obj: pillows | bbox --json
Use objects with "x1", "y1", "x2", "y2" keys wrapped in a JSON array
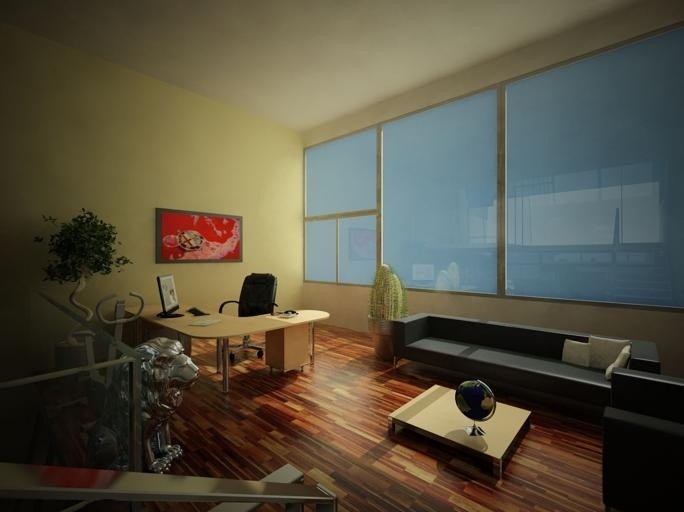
[
  {"x1": 588, "y1": 335, "x2": 631, "y2": 370},
  {"x1": 605, "y1": 341, "x2": 632, "y2": 380},
  {"x1": 561, "y1": 337, "x2": 592, "y2": 368}
]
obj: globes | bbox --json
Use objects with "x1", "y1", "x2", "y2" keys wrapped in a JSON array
[{"x1": 455, "y1": 379, "x2": 496, "y2": 436}]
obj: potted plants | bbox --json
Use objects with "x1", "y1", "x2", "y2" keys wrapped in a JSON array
[
  {"x1": 367, "y1": 264, "x2": 408, "y2": 362},
  {"x1": 33, "y1": 206, "x2": 135, "y2": 385}
]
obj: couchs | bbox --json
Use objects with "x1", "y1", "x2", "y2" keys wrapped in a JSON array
[{"x1": 392, "y1": 312, "x2": 660, "y2": 408}]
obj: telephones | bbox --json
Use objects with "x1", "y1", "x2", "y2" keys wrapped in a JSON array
[{"x1": 277, "y1": 310, "x2": 299, "y2": 318}]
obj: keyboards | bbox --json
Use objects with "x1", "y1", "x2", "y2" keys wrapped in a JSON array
[{"x1": 189, "y1": 308, "x2": 205, "y2": 316}]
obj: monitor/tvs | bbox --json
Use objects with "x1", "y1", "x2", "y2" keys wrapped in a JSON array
[{"x1": 157, "y1": 273, "x2": 184, "y2": 318}]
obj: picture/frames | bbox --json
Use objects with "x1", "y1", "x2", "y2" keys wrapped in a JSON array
[{"x1": 156, "y1": 208, "x2": 243, "y2": 263}]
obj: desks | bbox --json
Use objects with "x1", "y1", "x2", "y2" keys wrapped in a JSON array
[{"x1": 125, "y1": 302, "x2": 330, "y2": 394}]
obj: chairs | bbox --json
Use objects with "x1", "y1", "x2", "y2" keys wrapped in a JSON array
[
  {"x1": 219, "y1": 274, "x2": 277, "y2": 363},
  {"x1": 601, "y1": 366, "x2": 684, "y2": 512}
]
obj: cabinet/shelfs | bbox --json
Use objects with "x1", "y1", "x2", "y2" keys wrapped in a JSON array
[{"x1": 265, "y1": 322, "x2": 309, "y2": 378}]
